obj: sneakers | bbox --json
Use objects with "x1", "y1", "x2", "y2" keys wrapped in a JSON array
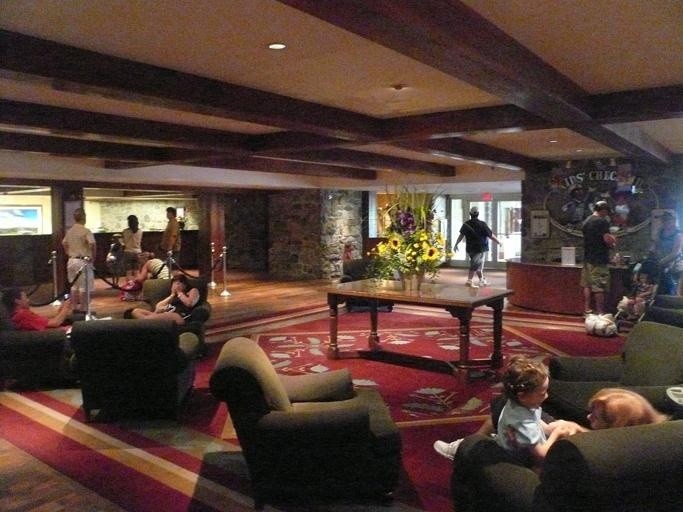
[
  {"x1": 432, "y1": 438, "x2": 465, "y2": 461},
  {"x1": 72, "y1": 309, "x2": 97, "y2": 316},
  {"x1": 583, "y1": 310, "x2": 594, "y2": 319},
  {"x1": 464, "y1": 280, "x2": 490, "y2": 289}
]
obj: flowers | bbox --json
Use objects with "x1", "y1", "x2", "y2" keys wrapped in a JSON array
[{"x1": 360, "y1": 180, "x2": 456, "y2": 283}]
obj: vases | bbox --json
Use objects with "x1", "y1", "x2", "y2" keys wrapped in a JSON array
[{"x1": 396, "y1": 268, "x2": 425, "y2": 291}]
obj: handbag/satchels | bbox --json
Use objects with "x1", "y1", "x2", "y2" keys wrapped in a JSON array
[{"x1": 584, "y1": 313, "x2": 616, "y2": 337}]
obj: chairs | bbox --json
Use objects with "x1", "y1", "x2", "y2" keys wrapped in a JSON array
[
  {"x1": 208, "y1": 336, "x2": 403, "y2": 511},
  {"x1": 0, "y1": 290, "x2": 93, "y2": 389},
  {"x1": 647, "y1": 293, "x2": 682, "y2": 328},
  {"x1": 123, "y1": 275, "x2": 213, "y2": 359},
  {"x1": 541, "y1": 318, "x2": 682, "y2": 429},
  {"x1": 338, "y1": 258, "x2": 394, "y2": 313},
  {"x1": 449, "y1": 416, "x2": 682, "y2": 511},
  {"x1": 69, "y1": 317, "x2": 200, "y2": 429}
]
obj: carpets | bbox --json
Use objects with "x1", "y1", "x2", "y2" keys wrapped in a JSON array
[{"x1": 0, "y1": 299, "x2": 628, "y2": 511}]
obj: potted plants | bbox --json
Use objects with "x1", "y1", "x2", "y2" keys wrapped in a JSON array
[{"x1": 175, "y1": 214, "x2": 187, "y2": 230}]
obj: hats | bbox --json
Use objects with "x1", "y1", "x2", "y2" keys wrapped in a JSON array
[{"x1": 469, "y1": 207, "x2": 479, "y2": 215}]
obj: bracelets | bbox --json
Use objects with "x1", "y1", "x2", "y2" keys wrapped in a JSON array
[{"x1": 177, "y1": 293, "x2": 185, "y2": 297}]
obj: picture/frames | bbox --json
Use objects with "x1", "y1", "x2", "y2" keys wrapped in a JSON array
[{"x1": 0, "y1": 203, "x2": 44, "y2": 236}]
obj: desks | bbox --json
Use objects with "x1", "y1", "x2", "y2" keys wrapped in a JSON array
[{"x1": 314, "y1": 276, "x2": 515, "y2": 384}]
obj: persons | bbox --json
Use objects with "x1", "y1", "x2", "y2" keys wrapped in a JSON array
[
  {"x1": 6, "y1": 287, "x2": 84, "y2": 331},
  {"x1": 123, "y1": 214, "x2": 143, "y2": 283},
  {"x1": 580, "y1": 198, "x2": 616, "y2": 319},
  {"x1": 134, "y1": 249, "x2": 174, "y2": 283},
  {"x1": 454, "y1": 206, "x2": 502, "y2": 286},
  {"x1": 161, "y1": 207, "x2": 182, "y2": 277},
  {"x1": 650, "y1": 211, "x2": 683, "y2": 296},
  {"x1": 434, "y1": 388, "x2": 666, "y2": 470},
  {"x1": 61, "y1": 208, "x2": 97, "y2": 315},
  {"x1": 132, "y1": 274, "x2": 201, "y2": 325},
  {"x1": 498, "y1": 354, "x2": 570, "y2": 466},
  {"x1": 106, "y1": 234, "x2": 124, "y2": 287},
  {"x1": 625, "y1": 268, "x2": 653, "y2": 320}
]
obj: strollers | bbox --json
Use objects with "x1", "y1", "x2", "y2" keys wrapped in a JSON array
[{"x1": 610, "y1": 254, "x2": 676, "y2": 333}]
